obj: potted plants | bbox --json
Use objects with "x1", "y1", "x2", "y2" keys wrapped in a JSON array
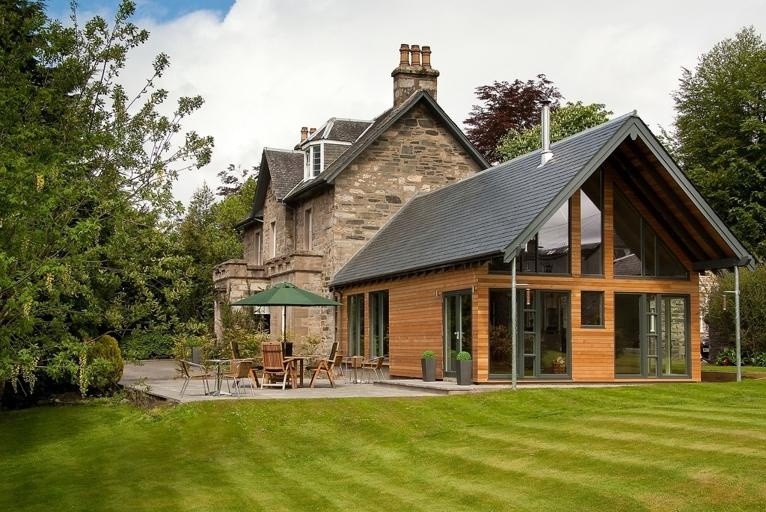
[
  {"x1": 421, "y1": 351, "x2": 437, "y2": 382},
  {"x1": 456, "y1": 352, "x2": 472, "y2": 385}
]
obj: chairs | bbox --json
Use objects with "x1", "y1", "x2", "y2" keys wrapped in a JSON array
[{"x1": 177, "y1": 342, "x2": 385, "y2": 399}]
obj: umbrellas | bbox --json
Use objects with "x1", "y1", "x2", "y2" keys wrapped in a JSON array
[{"x1": 227, "y1": 281, "x2": 345, "y2": 357}]
